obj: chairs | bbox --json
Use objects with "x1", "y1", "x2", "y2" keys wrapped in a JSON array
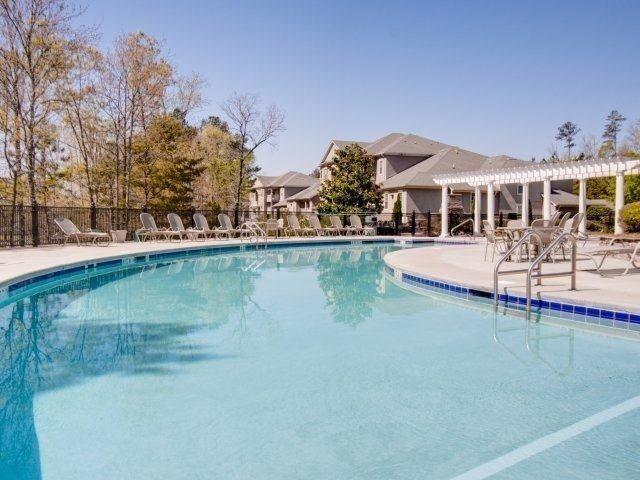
[
  {"x1": 132, "y1": 212, "x2": 378, "y2": 245},
  {"x1": 481, "y1": 209, "x2": 588, "y2": 278},
  {"x1": 49, "y1": 215, "x2": 112, "y2": 245}
]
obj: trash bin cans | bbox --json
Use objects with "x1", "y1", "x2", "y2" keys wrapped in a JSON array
[{"x1": 110, "y1": 230, "x2": 127, "y2": 243}]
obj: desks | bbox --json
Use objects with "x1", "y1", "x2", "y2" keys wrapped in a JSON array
[{"x1": 597, "y1": 232, "x2": 639, "y2": 276}]
